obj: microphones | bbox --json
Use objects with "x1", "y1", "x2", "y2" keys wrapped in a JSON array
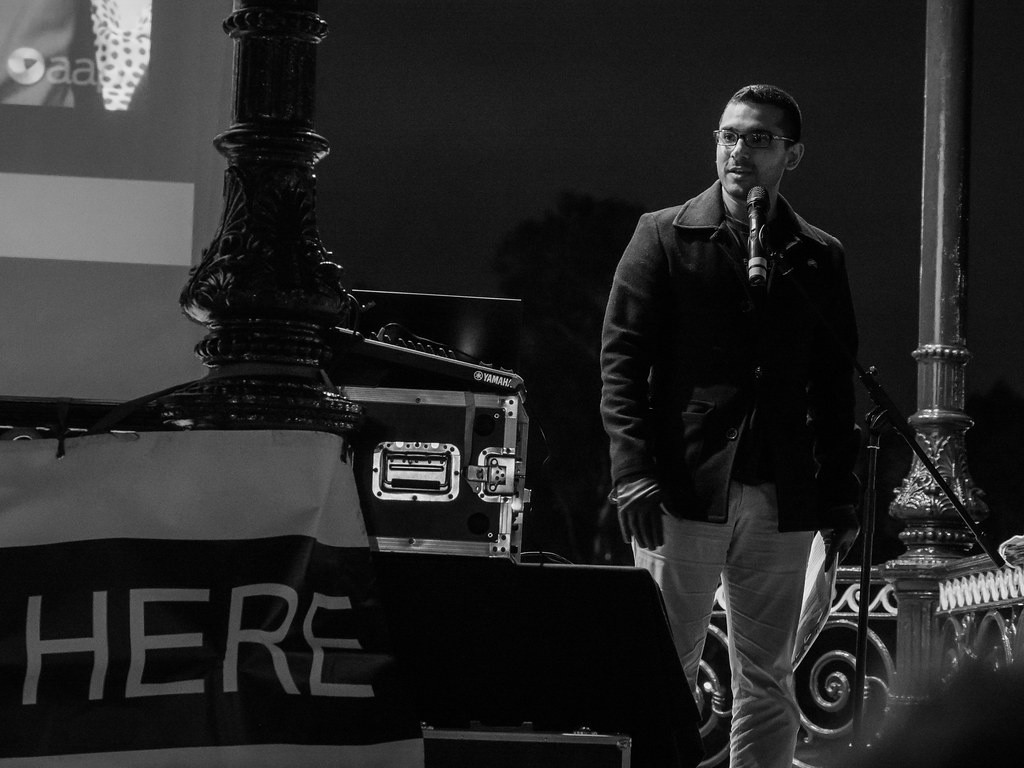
[{"x1": 745, "y1": 186, "x2": 771, "y2": 286}]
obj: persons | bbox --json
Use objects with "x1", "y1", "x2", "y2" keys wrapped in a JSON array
[{"x1": 599, "y1": 84, "x2": 862, "y2": 768}]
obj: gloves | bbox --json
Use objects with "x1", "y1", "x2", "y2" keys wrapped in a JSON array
[
  {"x1": 615, "y1": 473, "x2": 665, "y2": 551},
  {"x1": 813, "y1": 458, "x2": 863, "y2": 529}
]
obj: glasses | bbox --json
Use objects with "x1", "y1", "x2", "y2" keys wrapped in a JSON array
[{"x1": 712, "y1": 130, "x2": 796, "y2": 148}]
